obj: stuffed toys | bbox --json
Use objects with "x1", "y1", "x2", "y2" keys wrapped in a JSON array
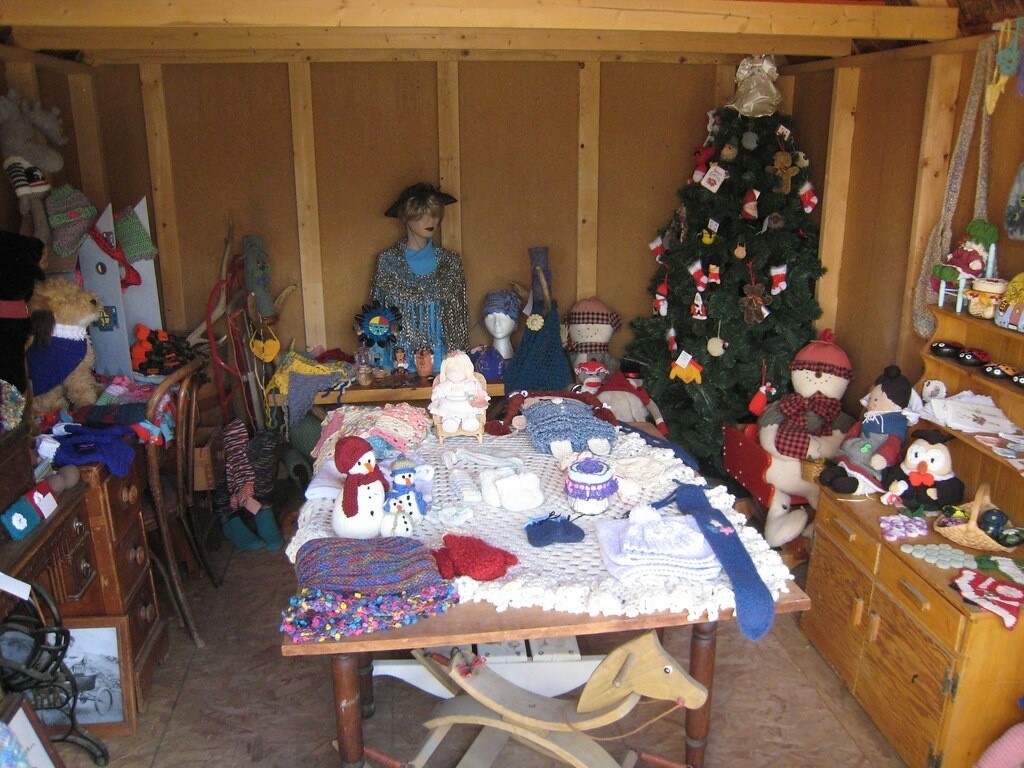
[
  {"x1": 330, "y1": 435, "x2": 426, "y2": 540},
  {"x1": 0, "y1": 96, "x2": 106, "y2": 496},
  {"x1": 560, "y1": 297, "x2": 622, "y2": 396},
  {"x1": 757, "y1": 331, "x2": 965, "y2": 548}
]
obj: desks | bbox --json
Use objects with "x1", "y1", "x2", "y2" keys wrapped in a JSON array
[
  {"x1": 280, "y1": 414, "x2": 812, "y2": 768},
  {"x1": 266, "y1": 373, "x2": 509, "y2": 426}
]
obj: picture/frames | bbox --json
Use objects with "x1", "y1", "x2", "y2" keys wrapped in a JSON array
[
  {"x1": 33, "y1": 611, "x2": 136, "y2": 737},
  {"x1": 2, "y1": 691, "x2": 68, "y2": 768}
]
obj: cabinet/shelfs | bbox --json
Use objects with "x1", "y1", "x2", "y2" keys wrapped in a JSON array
[
  {"x1": 1, "y1": 480, "x2": 108, "y2": 767},
  {"x1": 797, "y1": 302, "x2": 1024, "y2": 768},
  {"x1": 38, "y1": 429, "x2": 171, "y2": 716}
]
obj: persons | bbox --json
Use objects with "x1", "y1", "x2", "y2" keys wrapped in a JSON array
[
  {"x1": 428, "y1": 352, "x2": 491, "y2": 432},
  {"x1": 930, "y1": 219, "x2": 997, "y2": 281},
  {"x1": 369, "y1": 183, "x2": 471, "y2": 372},
  {"x1": 481, "y1": 290, "x2": 522, "y2": 359},
  {"x1": 392, "y1": 345, "x2": 410, "y2": 380}
]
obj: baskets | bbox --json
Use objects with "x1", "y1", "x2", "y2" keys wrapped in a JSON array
[{"x1": 932, "y1": 482, "x2": 1018, "y2": 554}]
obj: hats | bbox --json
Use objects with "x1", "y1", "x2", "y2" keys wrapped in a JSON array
[
  {"x1": 384, "y1": 183, "x2": 457, "y2": 218},
  {"x1": 482, "y1": 289, "x2": 521, "y2": 332}
]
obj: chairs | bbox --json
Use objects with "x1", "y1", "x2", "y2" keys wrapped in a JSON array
[{"x1": 141, "y1": 359, "x2": 222, "y2": 651}]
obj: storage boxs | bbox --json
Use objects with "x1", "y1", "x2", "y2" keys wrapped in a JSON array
[
  {"x1": 0, "y1": 422, "x2": 38, "y2": 518},
  {"x1": 187, "y1": 424, "x2": 222, "y2": 490}
]
